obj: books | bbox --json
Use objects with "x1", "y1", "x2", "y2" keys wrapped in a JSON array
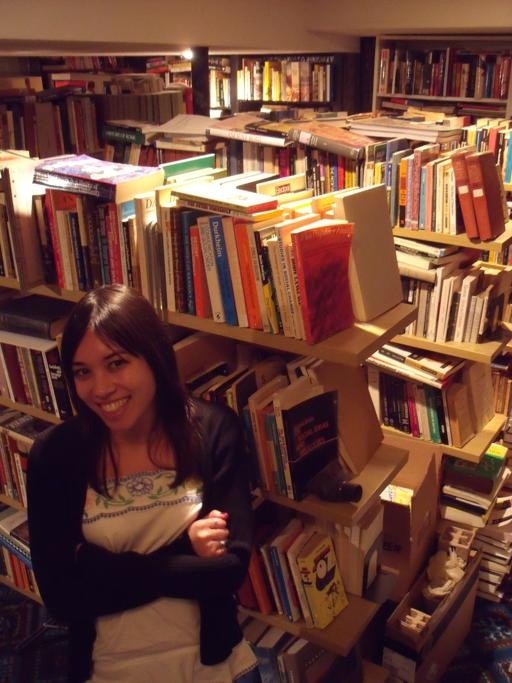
[{"x1": 2, "y1": 47, "x2": 511, "y2": 680}]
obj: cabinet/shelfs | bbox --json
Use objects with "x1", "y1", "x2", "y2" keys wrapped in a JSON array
[{"x1": 2, "y1": 31, "x2": 510, "y2": 676}]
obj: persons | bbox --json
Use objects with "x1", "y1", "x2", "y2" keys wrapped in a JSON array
[{"x1": 26, "y1": 284, "x2": 262, "y2": 678}]
